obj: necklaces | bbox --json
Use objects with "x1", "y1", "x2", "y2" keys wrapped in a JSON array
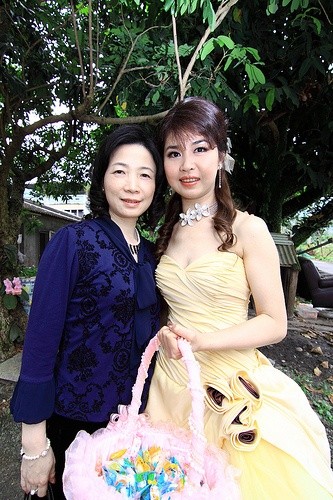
[
  {"x1": 20, "y1": 437, "x2": 50, "y2": 460},
  {"x1": 178, "y1": 202, "x2": 218, "y2": 227},
  {"x1": 127, "y1": 228, "x2": 140, "y2": 254}
]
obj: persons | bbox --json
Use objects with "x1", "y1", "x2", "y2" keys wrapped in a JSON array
[
  {"x1": 142, "y1": 97, "x2": 332, "y2": 494},
  {"x1": 8, "y1": 124, "x2": 159, "y2": 500}
]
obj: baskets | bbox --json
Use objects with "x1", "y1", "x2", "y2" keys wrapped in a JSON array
[{"x1": 62, "y1": 332, "x2": 238, "y2": 500}]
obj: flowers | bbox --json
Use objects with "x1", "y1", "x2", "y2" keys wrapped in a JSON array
[{"x1": 0, "y1": 276, "x2": 30, "y2": 310}]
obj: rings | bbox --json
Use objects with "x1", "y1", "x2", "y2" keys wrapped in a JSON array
[{"x1": 29, "y1": 489, "x2": 37, "y2": 495}]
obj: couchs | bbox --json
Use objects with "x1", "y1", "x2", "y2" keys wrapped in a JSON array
[{"x1": 296, "y1": 255, "x2": 333, "y2": 308}]
